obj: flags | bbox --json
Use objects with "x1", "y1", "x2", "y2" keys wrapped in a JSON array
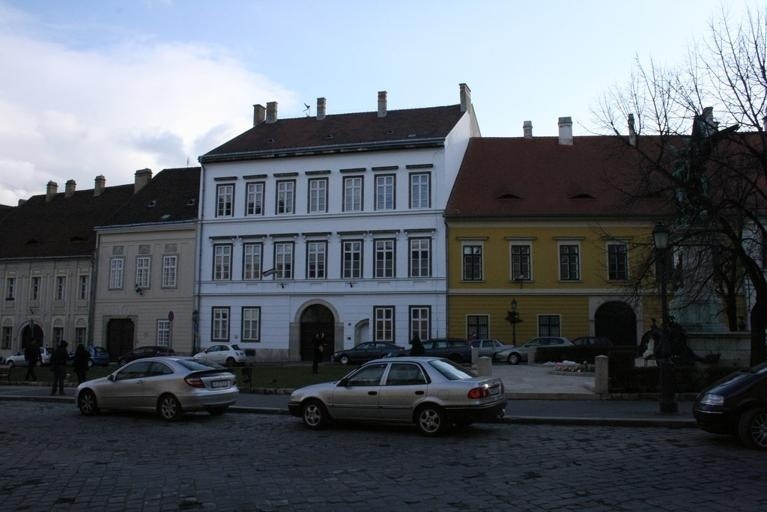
[{"x1": 262, "y1": 268, "x2": 278, "y2": 277}]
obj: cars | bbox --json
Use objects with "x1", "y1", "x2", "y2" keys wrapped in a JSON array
[
  {"x1": 6, "y1": 342, "x2": 243, "y2": 422},
  {"x1": 693, "y1": 359, "x2": 764, "y2": 445}
]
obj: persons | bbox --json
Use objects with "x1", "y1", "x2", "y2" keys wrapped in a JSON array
[
  {"x1": 651, "y1": 326, "x2": 684, "y2": 360},
  {"x1": 49, "y1": 340, "x2": 94, "y2": 396},
  {"x1": 311, "y1": 329, "x2": 324, "y2": 374},
  {"x1": 24, "y1": 336, "x2": 44, "y2": 381}
]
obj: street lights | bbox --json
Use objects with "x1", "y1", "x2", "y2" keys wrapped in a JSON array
[
  {"x1": 510, "y1": 297, "x2": 517, "y2": 345},
  {"x1": 653, "y1": 219, "x2": 677, "y2": 414}
]
